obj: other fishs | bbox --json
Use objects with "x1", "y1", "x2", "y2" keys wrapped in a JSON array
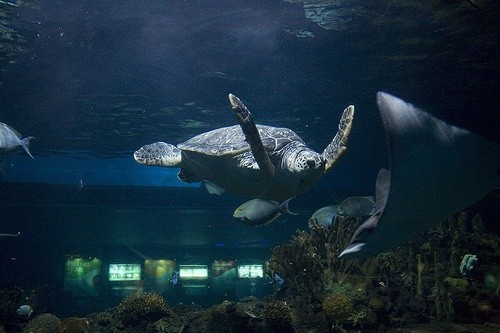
[
  {"x1": 0, "y1": 122, "x2": 38, "y2": 160},
  {"x1": 308, "y1": 196, "x2": 376, "y2": 230},
  {"x1": 232, "y1": 196, "x2": 299, "y2": 226}
]
